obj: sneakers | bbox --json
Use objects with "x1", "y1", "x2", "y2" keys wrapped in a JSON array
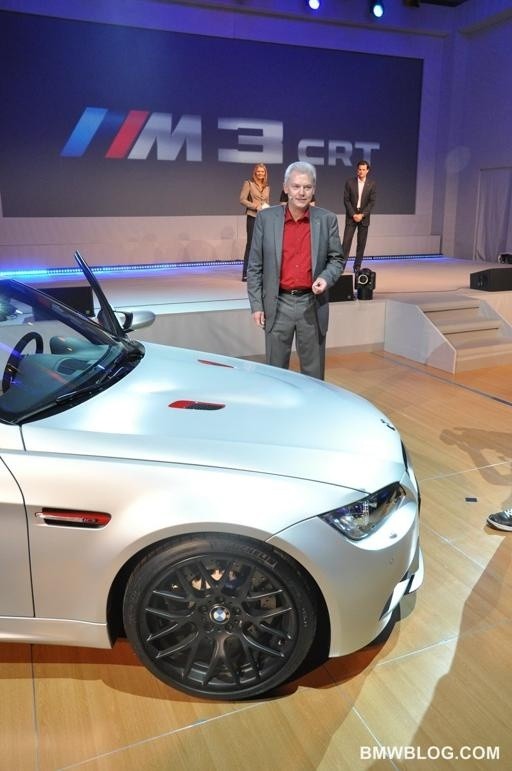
[{"x1": 488, "y1": 510, "x2": 511, "y2": 530}]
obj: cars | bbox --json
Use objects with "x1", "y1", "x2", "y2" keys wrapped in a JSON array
[{"x1": 0, "y1": 254, "x2": 425, "y2": 702}]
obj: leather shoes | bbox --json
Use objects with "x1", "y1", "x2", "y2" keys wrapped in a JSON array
[
  {"x1": 243, "y1": 277, "x2": 248, "y2": 281},
  {"x1": 355, "y1": 267, "x2": 360, "y2": 273}
]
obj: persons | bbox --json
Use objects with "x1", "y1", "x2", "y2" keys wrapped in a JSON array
[
  {"x1": 341, "y1": 160, "x2": 377, "y2": 273},
  {"x1": 485, "y1": 507, "x2": 512, "y2": 533},
  {"x1": 244, "y1": 162, "x2": 348, "y2": 382},
  {"x1": 277, "y1": 186, "x2": 317, "y2": 209},
  {"x1": 239, "y1": 162, "x2": 271, "y2": 282}
]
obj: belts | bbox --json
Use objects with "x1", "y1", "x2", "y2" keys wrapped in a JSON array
[
  {"x1": 280, "y1": 288, "x2": 312, "y2": 296},
  {"x1": 356, "y1": 208, "x2": 361, "y2": 211}
]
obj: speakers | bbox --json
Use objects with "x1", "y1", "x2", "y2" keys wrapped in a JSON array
[
  {"x1": 33, "y1": 286, "x2": 95, "y2": 321},
  {"x1": 470, "y1": 267, "x2": 512, "y2": 291},
  {"x1": 327, "y1": 275, "x2": 353, "y2": 302}
]
